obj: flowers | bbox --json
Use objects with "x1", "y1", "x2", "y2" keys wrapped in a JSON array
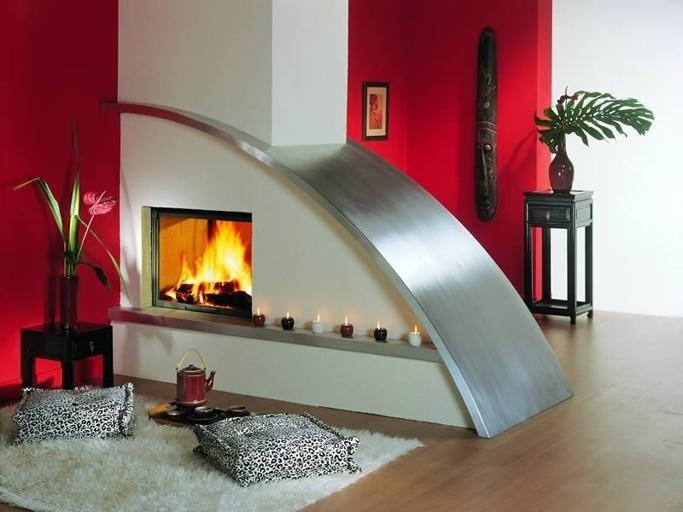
[{"x1": 12, "y1": 121, "x2": 133, "y2": 304}]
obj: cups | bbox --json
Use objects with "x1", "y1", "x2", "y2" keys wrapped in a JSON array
[
  {"x1": 229, "y1": 404, "x2": 248, "y2": 414},
  {"x1": 194, "y1": 405, "x2": 215, "y2": 418}
]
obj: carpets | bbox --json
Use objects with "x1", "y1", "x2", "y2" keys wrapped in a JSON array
[{"x1": 0, "y1": 384, "x2": 424, "y2": 511}]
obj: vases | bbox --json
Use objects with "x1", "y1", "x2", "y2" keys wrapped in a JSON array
[{"x1": 58, "y1": 276, "x2": 79, "y2": 332}]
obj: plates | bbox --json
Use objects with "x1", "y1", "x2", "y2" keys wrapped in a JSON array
[
  {"x1": 220, "y1": 410, "x2": 252, "y2": 419},
  {"x1": 185, "y1": 413, "x2": 217, "y2": 423}
]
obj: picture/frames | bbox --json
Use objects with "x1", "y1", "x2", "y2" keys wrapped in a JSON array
[{"x1": 362, "y1": 81, "x2": 390, "y2": 141}]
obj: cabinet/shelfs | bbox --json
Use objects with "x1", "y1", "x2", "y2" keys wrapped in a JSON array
[{"x1": 524, "y1": 189, "x2": 595, "y2": 327}]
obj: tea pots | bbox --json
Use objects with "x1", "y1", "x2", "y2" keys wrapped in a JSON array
[{"x1": 175, "y1": 348, "x2": 217, "y2": 407}]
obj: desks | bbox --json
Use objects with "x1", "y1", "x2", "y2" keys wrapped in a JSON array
[{"x1": 19, "y1": 321, "x2": 114, "y2": 390}]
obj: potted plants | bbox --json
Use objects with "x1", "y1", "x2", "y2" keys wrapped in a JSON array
[{"x1": 535, "y1": 86, "x2": 655, "y2": 192}]
